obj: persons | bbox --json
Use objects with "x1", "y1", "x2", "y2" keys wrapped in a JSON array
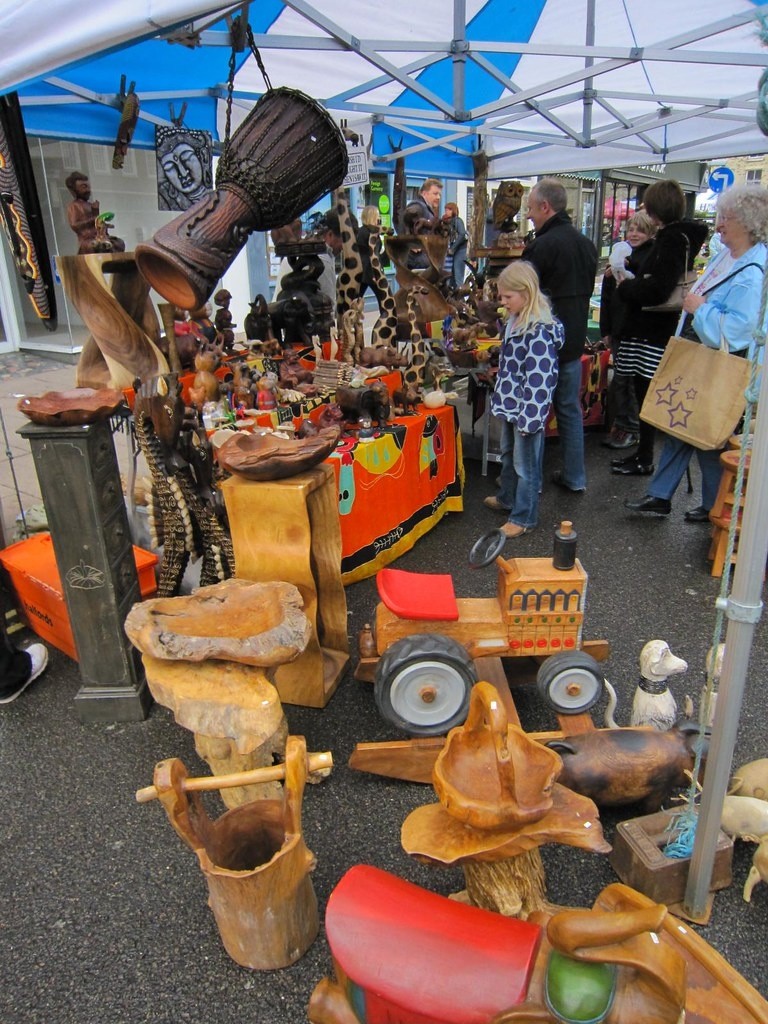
[
  {"x1": 271, "y1": 207, "x2": 358, "y2": 347},
  {"x1": 442, "y1": 201, "x2": 469, "y2": 291},
  {"x1": 404, "y1": 178, "x2": 444, "y2": 280},
  {"x1": 518, "y1": 179, "x2": 598, "y2": 493},
  {"x1": 357, "y1": 206, "x2": 383, "y2": 314},
  {"x1": 596, "y1": 180, "x2": 768, "y2": 521},
  {"x1": 481, "y1": 260, "x2": 567, "y2": 539},
  {"x1": 66, "y1": 172, "x2": 125, "y2": 254},
  {"x1": 277, "y1": 349, "x2": 318, "y2": 396},
  {"x1": 0, "y1": 612, "x2": 48, "y2": 704}
]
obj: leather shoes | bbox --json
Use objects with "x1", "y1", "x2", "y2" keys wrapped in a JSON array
[
  {"x1": 622, "y1": 495, "x2": 671, "y2": 514},
  {"x1": 611, "y1": 461, "x2": 654, "y2": 476},
  {"x1": 609, "y1": 453, "x2": 637, "y2": 466},
  {"x1": 685, "y1": 506, "x2": 710, "y2": 522}
]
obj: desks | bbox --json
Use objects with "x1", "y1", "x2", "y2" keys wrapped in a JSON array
[{"x1": 123, "y1": 316, "x2": 610, "y2": 583}]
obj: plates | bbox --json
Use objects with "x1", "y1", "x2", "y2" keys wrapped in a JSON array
[{"x1": 16, "y1": 387, "x2": 126, "y2": 426}]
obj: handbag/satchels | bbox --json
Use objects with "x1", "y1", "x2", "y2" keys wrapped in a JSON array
[
  {"x1": 638, "y1": 314, "x2": 760, "y2": 450},
  {"x1": 642, "y1": 232, "x2": 698, "y2": 311},
  {"x1": 680, "y1": 263, "x2": 764, "y2": 359}
]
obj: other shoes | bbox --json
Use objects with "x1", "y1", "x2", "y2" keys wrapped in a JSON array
[
  {"x1": 499, "y1": 522, "x2": 535, "y2": 538},
  {"x1": 483, "y1": 496, "x2": 507, "y2": 509},
  {"x1": 609, "y1": 432, "x2": 640, "y2": 448},
  {"x1": 600, "y1": 430, "x2": 626, "y2": 443},
  {"x1": 0, "y1": 643, "x2": 48, "y2": 704},
  {"x1": 553, "y1": 470, "x2": 585, "y2": 494},
  {"x1": 496, "y1": 475, "x2": 542, "y2": 495}
]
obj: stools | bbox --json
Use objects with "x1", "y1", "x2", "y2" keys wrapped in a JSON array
[{"x1": 706, "y1": 433, "x2": 754, "y2": 578}]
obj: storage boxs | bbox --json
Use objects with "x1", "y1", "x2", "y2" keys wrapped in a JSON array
[{"x1": 0, "y1": 531, "x2": 159, "y2": 664}]
